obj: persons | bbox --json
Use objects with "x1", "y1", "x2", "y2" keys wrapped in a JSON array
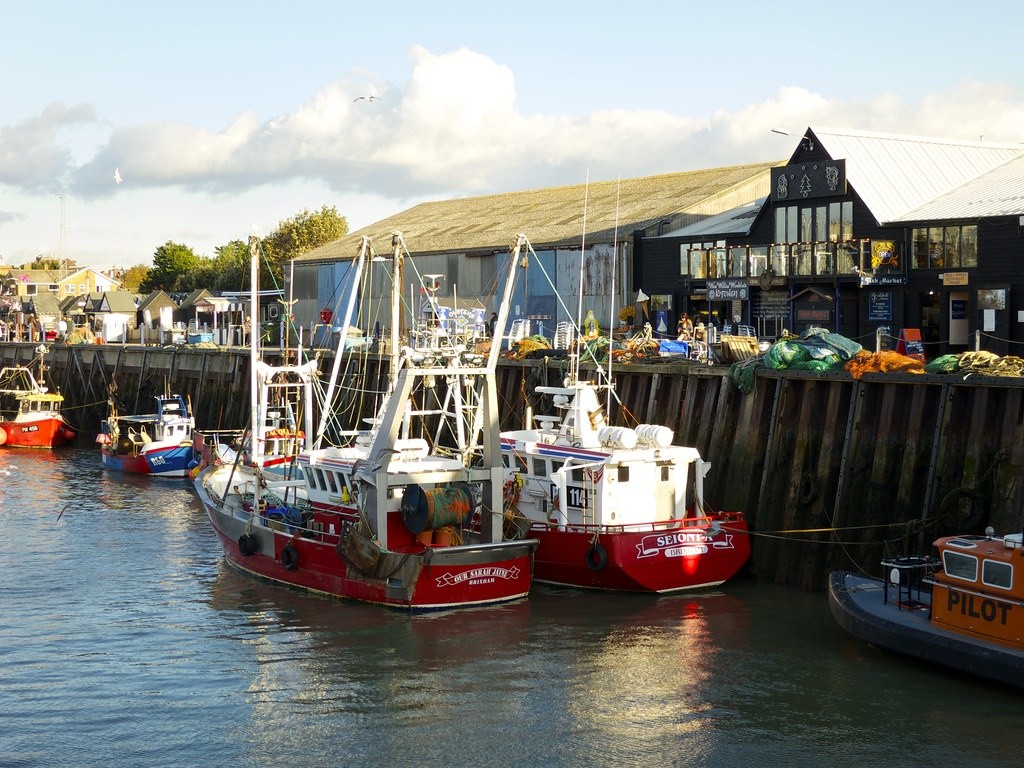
[
  {"x1": 489, "y1": 312, "x2": 498, "y2": 337},
  {"x1": 695, "y1": 317, "x2": 707, "y2": 341},
  {"x1": 677, "y1": 312, "x2": 694, "y2": 340},
  {"x1": 584, "y1": 310, "x2": 599, "y2": 337}
]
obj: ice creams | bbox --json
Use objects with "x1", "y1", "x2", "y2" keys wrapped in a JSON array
[{"x1": 636, "y1": 289, "x2": 650, "y2": 319}]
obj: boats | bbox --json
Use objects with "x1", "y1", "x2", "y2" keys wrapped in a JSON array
[
  {"x1": 829, "y1": 525, "x2": 1023, "y2": 699},
  {"x1": 188, "y1": 332, "x2": 215, "y2": 344},
  {"x1": 187, "y1": 231, "x2": 544, "y2": 612},
  {"x1": 95, "y1": 372, "x2": 210, "y2": 478},
  {"x1": 399, "y1": 344, "x2": 467, "y2": 363},
  {"x1": 0, "y1": 353, "x2": 82, "y2": 449},
  {"x1": 461, "y1": 231, "x2": 754, "y2": 597},
  {"x1": 39, "y1": 331, "x2": 57, "y2": 342}
]
